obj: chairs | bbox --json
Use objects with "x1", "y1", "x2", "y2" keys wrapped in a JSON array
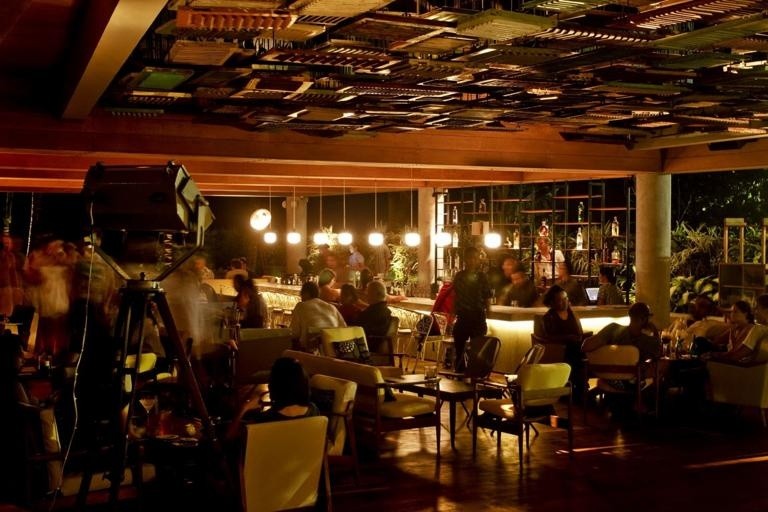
[
  {"x1": 309, "y1": 374, "x2": 357, "y2": 487},
  {"x1": 387, "y1": 305, "x2": 448, "y2": 375},
  {"x1": 319, "y1": 326, "x2": 405, "y2": 377},
  {"x1": 582, "y1": 344, "x2": 655, "y2": 424},
  {"x1": 238, "y1": 415, "x2": 332, "y2": 512},
  {"x1": 435, "y1": 336, "x2": 501, "y2": 417},
  {"x1": 258, "y1": 292, "x2": 302, "y2": 329},
  {"x1": 476, "y1": 362, "x2": 573, "y2": 467},
  {"x1": 531, "y1": 314, "x2": 593, "y2": 363}
]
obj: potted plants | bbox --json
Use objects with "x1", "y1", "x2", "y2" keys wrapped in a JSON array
[{"x1": 387, "y1": 244, "x2": 412, "y2": 297}]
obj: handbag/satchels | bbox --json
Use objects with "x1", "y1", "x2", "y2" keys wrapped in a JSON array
[{"x1": 431, "y1": 269, "x2": 464, "y2": 314}]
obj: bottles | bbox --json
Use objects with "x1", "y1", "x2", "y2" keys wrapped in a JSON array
[
  {"x1": 512, "y1": 228, "x2": 519, "y2": 249},
  {"x1": 489, "y1": 287, "x2": 496, "y2": 305},
  {"x1": 577, "y1": 202, "x2": 584, "y2": 221},
  {"x1": 610, "y1": 245, "x2": 620, "y2": 265},
  {"x1": 611, "y1": 216, "x2": 618, "y2": 237},
  {"x1": 478, "y1": 198, "x2": 485, "y2": 213},
  {"x1": 575, "y1": 227, "x2": 582, "y2": 250},
  {"x1": 452, "y1": 230, "x2": 458, "y2": 248},
  {"x1": 452, "y1": 206, "x2": 458, "y2": 224},
  {"x1": 537, "y1": 221, "x2": 549, "y2": 237}
]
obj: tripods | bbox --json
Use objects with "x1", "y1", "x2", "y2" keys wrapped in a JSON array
[{"x1": 68, "y1": 284, "x2": 220, "y2": 507}]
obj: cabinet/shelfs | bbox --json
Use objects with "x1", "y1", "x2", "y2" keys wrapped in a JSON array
[{"x1": 720, "y1": 264, "x2": 766, "y2": 324}]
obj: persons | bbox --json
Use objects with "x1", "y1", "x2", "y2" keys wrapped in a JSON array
[
  {"x1": 451, "y1": 247, "x2": 494, "y2": 380},
  {"x1": 427, "y1": 279, "x2": 458, "y2": 336},
  {"x1": 371, "y1": 243, "x2": 391, "y2": 277},
  {"x1": 224, "y1": 259, "x2": 248, "y2": 279},
  {"x1": 661, "y1": 294, "x2": 731, "y2": 355},
  {"x1": 596, "y1": 267, "x2": 623, "y2": 305},
  {"x1": 336, "y1": 284, "x2": 362, "y2": 324},
  {"x1": 534, "y1": 237, "x2": 566, "y2": 286},
  {"x1": 315, "y1": 245, "x2": 335, "y2": 264},
  {"x1": 580, "y1": 303, "x2": 661, "y2": 395},
  {"x1": 232, "y1": 275, "x2": 267, "y2": 329},
  {"x1": 195, "y1": 251, "x2": 214, "y2": 280},
  {"x1": 700, "y1": 294, "x2": 768, "y2": 364},
  {"x1": 496, "y1": 257, "x2": 537, "y2": 305},
  {"x1": 714, "y1": 299, "x2": 757, "y2": 366},
  {"x1": 239, "y1": 256, "x2": 258, "y2": 278},
  {"x1": 214, "y1": 358, "x2": 320, "y2": 498},
  {"x1": 287, "y1": 282, "x2": 348, "y2": 354},
  {"x1": 541, "y1": 285, "x2": 584, "y2": 361},
  {"x1": 344, "y1": 242, "x2": 364, "y2": 282},
  {"x1": 351, "y1": 280, "x2": 392, "y2": 351},
  {"x1": 325, "y1": 253, "x2": 348, "y2": 281},
  {"x1": 318, "y1": 269, "x2": 340, "y2": 303},
  {"x1": 0, "y1": 233, "x2": 117, "y2": 333},
  {"x1": 354, "y1": 268, "x2": 408, "y2": 303}
]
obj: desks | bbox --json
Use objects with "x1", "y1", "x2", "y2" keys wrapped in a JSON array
[
  {"x1": 383, "y1": 374, "x2": 502, "y2": 450},
  {"x1": 655, "y1": 355, "x2": 706, "y2": 417}
]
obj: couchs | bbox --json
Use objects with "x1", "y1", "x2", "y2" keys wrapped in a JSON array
[
  {"x1": 685, "y1": 316, "x2": 768, "y2": 427},
  {"x1": 281, "y1": 350, "x2": 441, "y2": 459}
]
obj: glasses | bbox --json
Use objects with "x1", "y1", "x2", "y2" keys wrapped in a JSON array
[{"x1": 645, "y1": 312, "x2": 653, "y2": 317}]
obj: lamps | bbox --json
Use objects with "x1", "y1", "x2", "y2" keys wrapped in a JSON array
[
  {"x1": 433, "y1": 231, "x2": 452, "y2": 247},
  {"x1": 312, "y1": 178, "x2": 328, "y2": 244},
  {"x1": 484, "y1": 231, "x2": 502, "y2": 248},
  {"x1": 367, "y1": 181, "x2": 383, "y2": 247},
  {"x1": 404, "y1": 168, "x2": 420, "y2": 247},
  {"x1": 250, "y1": 208, "x2": 271, "y2": 277},
  {"x1": 286, "y1": 186, "x2": 302, "y2": 244},
  {"x1": 263, "y1": 185, "x2": 277, "y2": 244},
  {"x1": 337, "y1": 179, "x2": 352, "y2": 245}
]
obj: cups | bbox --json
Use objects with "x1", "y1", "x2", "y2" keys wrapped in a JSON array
[{"x1": 510, "y1": 301, "x2": 518, "y2": 308}]
